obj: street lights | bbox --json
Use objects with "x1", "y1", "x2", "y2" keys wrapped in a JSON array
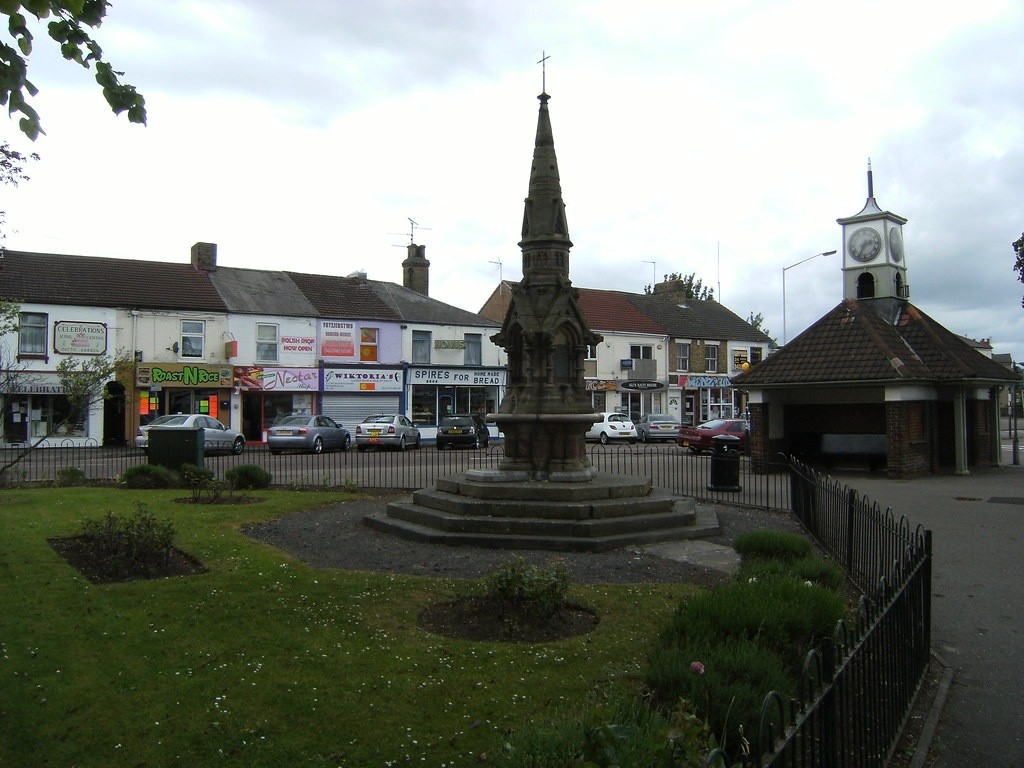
[{"x1": 782, "y1": 250, "x2": 838, "y2": 345}]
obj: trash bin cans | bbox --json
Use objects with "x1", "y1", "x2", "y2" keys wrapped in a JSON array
[{"x1": 706, "y1": 433, "x2": 742, "y2": 493}]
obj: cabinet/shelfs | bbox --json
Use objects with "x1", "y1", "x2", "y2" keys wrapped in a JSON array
[{"x1": 412, "y1": 412, "x2": 435, "y2": 425}]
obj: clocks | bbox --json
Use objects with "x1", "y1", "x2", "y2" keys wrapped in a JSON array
[
  {"x1": 848, "y1": 226, "x2": 883, "y2": 261},
  {"x1": 890, "y1": 226, "x2": 904, "y2": 263}
]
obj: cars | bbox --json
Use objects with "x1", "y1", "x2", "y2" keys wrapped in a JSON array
[
  {"x1": 676, "y1": 418, "x2": 750, "y2": 455},
  {"x1": 355, "y1": 414, "x2": 421, "y2": 451},
  {"x1": 134, "y1": 414, "x2": 246, "y2": 455},
  {"x1": 636, "y1": 413, "x2": 680, "y2": 443},
  {"x1": 435, "y1": 413, "x2": 491, "y2": 449},
  {"x1": 266, "y1": 414, "x2": 350, "y2": 454},
  {"x1": 739, "y1": 412, "x2": 750, "y2": 429},
  {"x1": 585, "y1": 412, "x2": 638, "y2": 444}
]
